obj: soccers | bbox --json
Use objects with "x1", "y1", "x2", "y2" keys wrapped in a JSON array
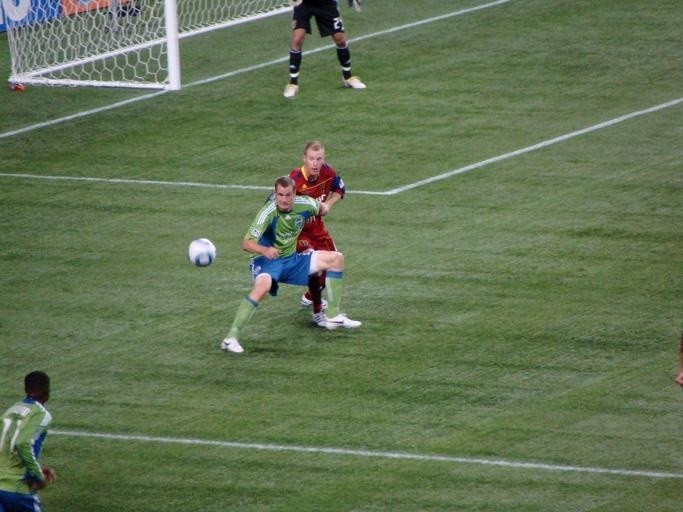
[{"x1": 188, "y1": 237, "x2": 214, "y2": 266}]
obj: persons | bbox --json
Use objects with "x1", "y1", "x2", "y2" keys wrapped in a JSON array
[
  {"x1": 287, "y1": 141, "x2": 345, "y2": 310},
  {"x1": 0, "y1": 371, "x2": 58, "y2": 512},
  {"x1": 220, "y1": 176, "x2": 363, "y2": 354},
  {"x1": 282, "y1": 0, "x2": 367, "y2": 98}
]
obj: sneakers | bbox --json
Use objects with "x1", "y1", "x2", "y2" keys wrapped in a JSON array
[
  {"x1": 221, "y1": 337, "x2": 245, "y2": 353},
  {"x1": 300, "y1": 293, "x2": 362, "y2": 331},
  {"x1": 342, "y1": 75, "x2": 367, "y2": 89},
  {"x1": 283, "y1": 84, "x2": 298, "y2": 98}
]
